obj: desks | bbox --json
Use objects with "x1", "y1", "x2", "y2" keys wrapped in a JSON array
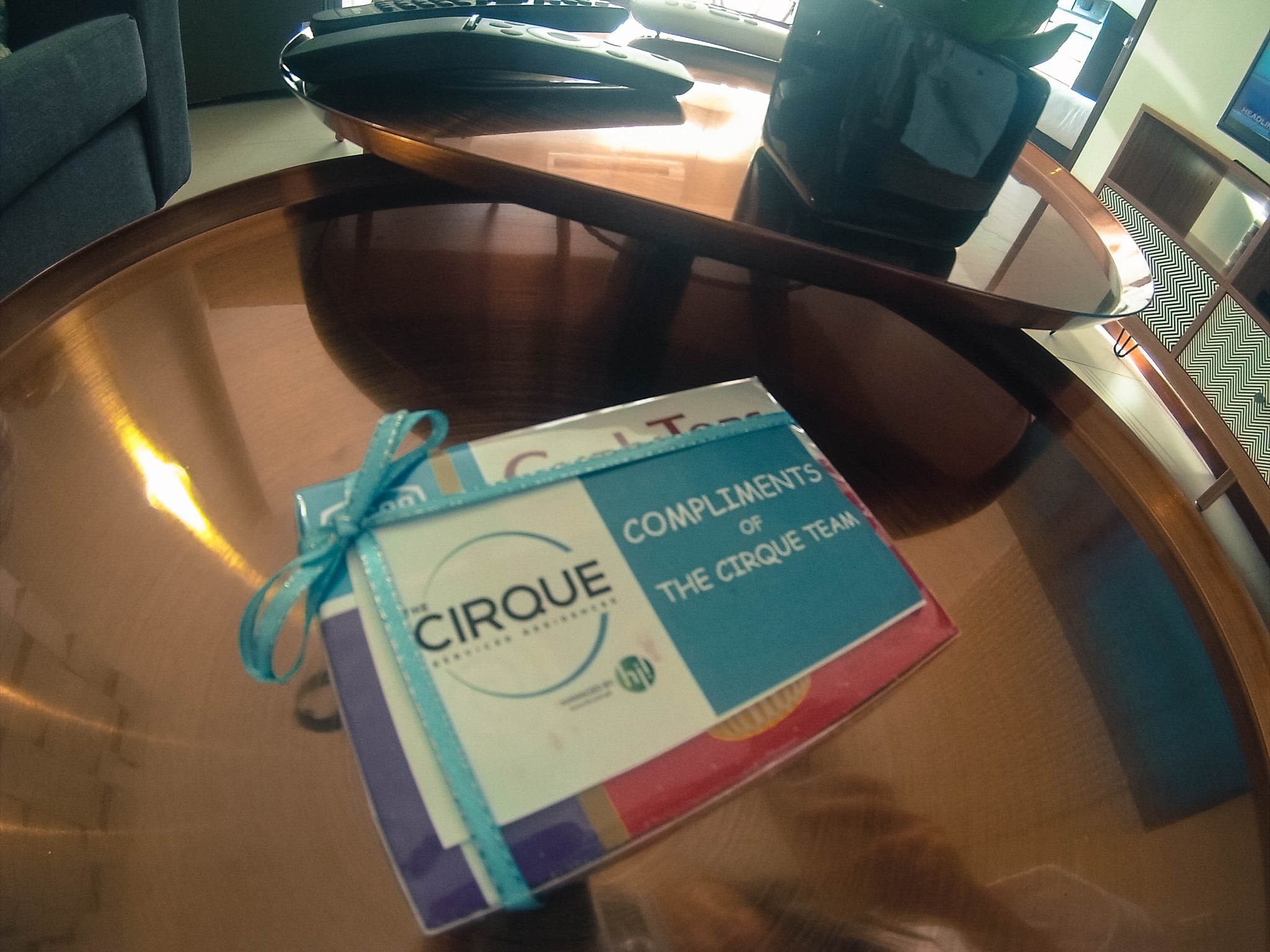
[
  {"x1": 277, "y1": 23, "x2": 1155, "y2": 331},
  {"x1": 0, "y1": 150, "x2": 1270, "y2": 952}
]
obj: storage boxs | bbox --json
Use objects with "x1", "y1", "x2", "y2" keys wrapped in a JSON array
[{"x1": 294, "y1": 377, "x2": 960, "y2": 938}]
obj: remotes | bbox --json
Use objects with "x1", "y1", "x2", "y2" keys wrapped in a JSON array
[
  {"x1": 308, "y1": 1, "x2": 629, "y2": 39},
  {"x1": 284, "y1": 13, "x2": 692, "y2": 96},
  {"x1": 628, "y1": 0, "x2": 791, "y2": 62}
]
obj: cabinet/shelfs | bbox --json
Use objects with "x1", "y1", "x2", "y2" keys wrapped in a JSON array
[{"x1": 1092, "y1": 103, "x2": 1270, "y2": 534}]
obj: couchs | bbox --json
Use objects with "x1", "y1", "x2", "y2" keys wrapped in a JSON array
[{"x1": 0, "y1": 0, "x2": 192, "y2": 297}]
